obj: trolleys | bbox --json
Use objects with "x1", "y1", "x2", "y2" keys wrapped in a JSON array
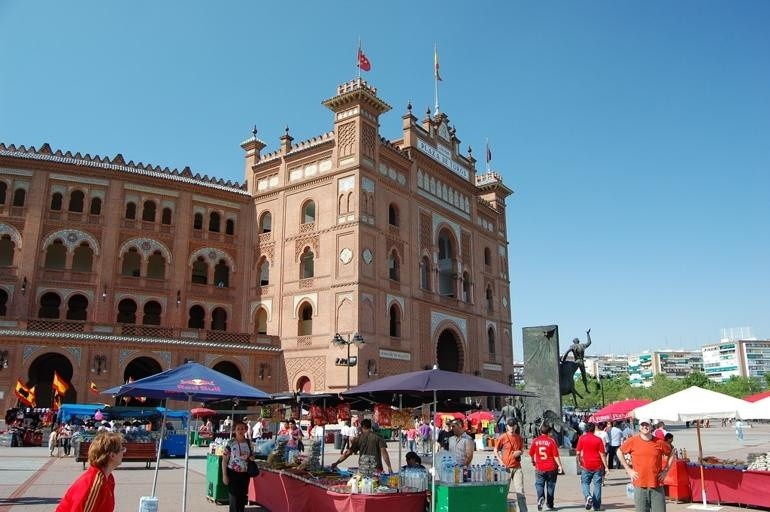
[{"x1": 204, "y1": 453, "x2": 231, "y2": 507}]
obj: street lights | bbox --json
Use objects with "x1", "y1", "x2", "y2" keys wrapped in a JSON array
[{"x1": 331, "y1": 330, "x2": 365, "y2": 391}]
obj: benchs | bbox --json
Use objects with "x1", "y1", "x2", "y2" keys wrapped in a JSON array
[{"x1": 75, "y1": 441, "x2": 158, "y2": 470}]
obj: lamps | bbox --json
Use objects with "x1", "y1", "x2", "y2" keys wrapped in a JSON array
[
  {"x1": 20, "y1": 277, "x2": 27, "y2": 296},
  {"x1": 0, "y1": 351, "x2": 9, "y2": 372},
  {"x1": 90, "y1": 354, "x2": 107, "y2": 376},
  {"x1": 368, "y1": 359, "x2": 377, "y2": 377},
  {"x1": 101, "y1": 285, "x2": 107, "y2": 301},
  {"x1": 175, "y1": 291, "x2": 181, "y2": 308},
  {"x1": 258, "y1": 364, "x2": 271, "y2": 380}
]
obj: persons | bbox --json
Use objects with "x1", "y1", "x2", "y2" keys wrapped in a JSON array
[
  {"x1": 528, "y1": 424, "x2": 565, "y2": 512},
  {"x1": 220, "y1": 419, "x2": 256, "y2": 512},
  {"x1": 615, "y1": 416, "x2": 675, "y2": 512},
  {"x1": 447, "y1": 417, "x2": 474, "y2": 467},
  {"x1": 559, "y1": 327, "x2": 592, "y2": 394},
  {"x1": 331, "y1": 418, "x2": 394, "y2": 474},
  {"x1": 48, "y1": 426, "x2": 56, "y2": 457},
  {"x1": 494, "y1": 418, "x2": 530, "y2": 512},
  {"x1": 54, "y1": 431, "x2": 126, "y2": 512},
  {"x1": 494, "y1": 395, "x2": 517, "y2": 426},
  {"x1": 222, "y1": 415, "x2": 476, "y2": 457},
  {"x1": 400, "y1": 451, "x2": 428, "y2": 471},
  {"x1": 683, "y1": 414, "x2": 769, "y2": 430},
  {"x1": 51, "y1": 409, "x2": 57, "y2": 433},
  {"x1": 562, "y1": 411, "x2": 680, "y2": 488},
  {"x1": 278, "y1": 418, "x2": 290, "y2": 464},
  {"x1": 63, "y1": 424, "x2": 74, "y2": 458},
  {"x1": 576, "y1": 421, "x2": 610, "y2": 511},
  {"x1": 205, "y1": 416, "x2": 214, "y2": 433},
  {"x1": 286, "y1": 419, "x2": 304, "y2": 464}
]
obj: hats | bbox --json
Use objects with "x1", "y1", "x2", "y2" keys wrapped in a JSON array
[
  {"x1": 507, "y1": 419, "x2": 516, "y2": 426},
  {"x1": 639, "y1": 418, "x2": 651, "y2": 425}
]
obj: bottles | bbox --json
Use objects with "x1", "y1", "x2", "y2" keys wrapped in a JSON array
[
  {"x1": 380, "y1": 474, "x2": 390, "y2": 485},
  {"x1": 679, "y1": 448, "x2": 687, "y2": 460},
  {"x1": 439, "y1": 454, "x2": 508, "y2": 484},
  {"x1": 210, "y1": 437, "x2": 231, "y2": 457},
  {"x1": 627, "y1": 482, "x2": 634, "y2": 498},
  {"x1": 350, "y1": 475, "x2": 374, "y2": 494},
  {"x1": 255, "y1": 437, "x2": 271, "y2": 452},
  {"x1": 399, "y1": 467, "x2": 429, "y2": 493}
]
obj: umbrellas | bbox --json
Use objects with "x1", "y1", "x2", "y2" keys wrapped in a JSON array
[
  {"x1": 115, "y1": 359, "x2": 276, "y2": 511},
  {"x1": 271, "y1": 391, "x2": 373, "y2": 411},
  {"x1": 99, "y1": 380, "x2": 210, "y2": 496},
  {"x1": 586, "y1": 398, "x2": 653, "y2": 430},
  {"x1": 338, "y1": 362, "x2": 539, "y2": 512},
  {"x1": 736, "y1": 394, "x2": 770, "y2": 421},
  {"x1": 625, "y1": 383, "x2": 753, "y2": 506}
]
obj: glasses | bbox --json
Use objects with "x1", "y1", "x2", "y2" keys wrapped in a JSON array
[{"x1": 119, "y1": 447, "x2": 126, "y2": 453}]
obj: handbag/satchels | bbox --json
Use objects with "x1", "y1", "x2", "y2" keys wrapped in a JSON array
[{"x1": 247, "y1": 461, "x2": 259, "y2": 478}]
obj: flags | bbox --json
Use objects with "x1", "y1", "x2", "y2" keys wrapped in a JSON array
[
  {"x1": 14, "y1": 377, "x2": 35, "y2": 408},
  {"x1": 486, "y1": 144, "x2": 492, "y2": 161},
  {"x1": 51, "y1": 388, "x2": 62, "y2": 412},
  {"x1": 89, "y1": 379, "x2": 100, "y2": 395},
  {"x1": 119, "y1": 375, "x2": 148, "y2": 404},
  {"x1": 52, "y1": 370, "x2": 70, "y2": 397},
  {"x1": 26, "y1": 384, "x2": 37, "y2": 410},
  {"x1": 356, "y1": 48, "x2": 372, "y2": 72},
  {"x1": 433, "y1": 50, "x2": 444, "y2": 82}
]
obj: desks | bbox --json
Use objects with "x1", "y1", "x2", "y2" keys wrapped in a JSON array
[
  {"x1": 246, "y1": 456, "x2": 429, "y2": 511},
  {"x1": 688, "y1": 457, "x2": 770, "y2": 509}
]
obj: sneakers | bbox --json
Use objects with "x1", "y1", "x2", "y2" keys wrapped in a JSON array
[
  {"x1": 546, "y1": 507, "x2": 557, "y2": 511},
  {"x1": 586, "y1": 496, "x2": 593, "y2": 510},
  {"x1": 538, "y1": 497, "x2": 545, "y2": 510}
]
